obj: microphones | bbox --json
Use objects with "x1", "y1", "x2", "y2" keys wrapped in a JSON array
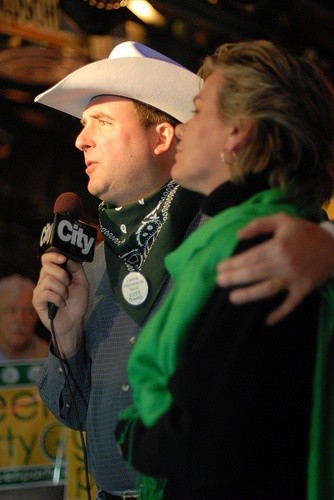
[{"x1": 38, "y1": 192, "x2": 97, "y2": 319}]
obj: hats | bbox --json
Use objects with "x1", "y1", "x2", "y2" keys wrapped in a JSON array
[{"x1": 33, "y1": 40, "x2": 206, "y2": 125}]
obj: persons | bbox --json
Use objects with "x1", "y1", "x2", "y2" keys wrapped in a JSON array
[
  {"x1": 113, "y1": 40, "x2": 334, "y2": 500},
  {"x1": 31, "y1": 40, "x2": 334, "y2": 500},
  {"x1": 0, "y1": 274, "x2": 50, "y2": 360}
]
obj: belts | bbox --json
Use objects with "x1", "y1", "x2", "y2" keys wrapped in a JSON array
[{"x1": 96, "y1": 490, "x2": 138, "y2": 500}]
